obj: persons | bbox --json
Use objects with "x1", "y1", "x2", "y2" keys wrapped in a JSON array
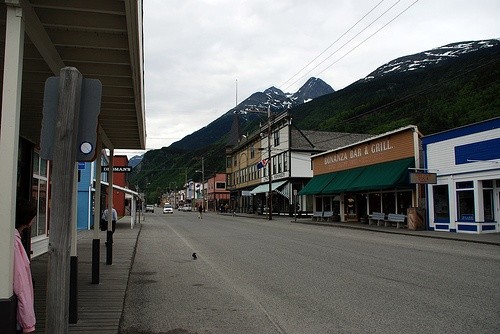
[
  {"x1": 102, "y1": 203, "x2": 117, "y2": 244},
  {"x1": 198, "y1": 205, "x2": 204, "y2": 219},
  {"x1": 14, "y1": 199, "x2": 36, "y2": 334},
  {"x1": 221, "y1": 203, "x2": 228, "y2": 213}
]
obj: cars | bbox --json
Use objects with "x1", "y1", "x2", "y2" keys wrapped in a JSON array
[
  {"x1": 163, "y1": 205, "x2": 174, "y2": 214},
  {"x1": 178, "y1": 203, "x2": 192, "y2": 212},
  {"x1": 145, "y1": 204, "x2": 155, "y2": 213}
]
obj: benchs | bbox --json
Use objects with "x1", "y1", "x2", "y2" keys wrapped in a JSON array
[
  {"x1": 368, "y1": 212, "x2": 385, "y2": 226},
  {"x1": 311, "y1": 211, "x2": 323, "y2": 222},
  {"x1": 384, "y1": 214, "x2": 405, "y2": 228},
  {"x1": 322, "y1": 211, "x2": 334, "y2": 221}
]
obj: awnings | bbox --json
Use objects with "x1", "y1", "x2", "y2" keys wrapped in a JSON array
[
  {"x1": 297, "y1": 158, "x2": 414, "y2": 195},
  {"x1": 250, "y1": 180, "x2": 288, "y2": 194}
]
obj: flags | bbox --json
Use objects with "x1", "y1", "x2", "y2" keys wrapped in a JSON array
[{"x1": 257, "y1": 158, "x2": 270, "y2": 169}]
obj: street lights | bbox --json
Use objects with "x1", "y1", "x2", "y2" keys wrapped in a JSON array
[
  {"x1": 195, "y1": 170, "x2": 206, "y2": 213},
  {"x1": 145, "y1": 182, "x2": 151, "y2": 204},
  {"x1": 259, "y1": 133, "x2": 273, "y2": 220}
]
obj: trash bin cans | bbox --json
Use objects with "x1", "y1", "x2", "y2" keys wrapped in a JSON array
[{"x1": 407, "y1": 208, "x2": 425, "y2": 230}]
